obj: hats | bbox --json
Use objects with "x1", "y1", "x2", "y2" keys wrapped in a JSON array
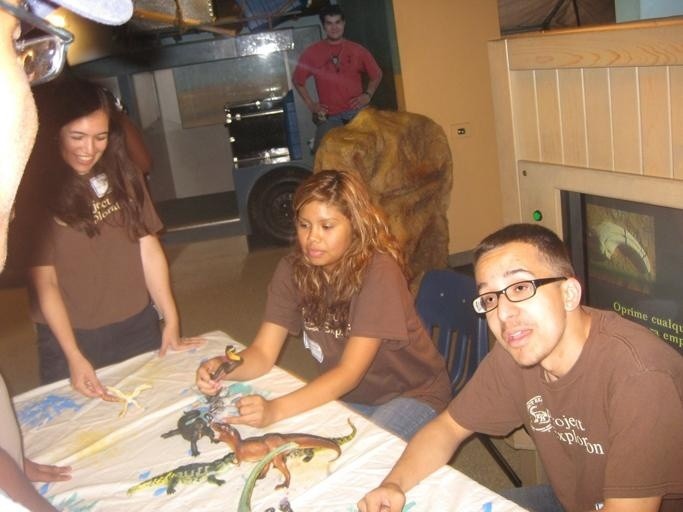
[{"x1": 21, "y1": 0, "x2": 133, "y2": 36}]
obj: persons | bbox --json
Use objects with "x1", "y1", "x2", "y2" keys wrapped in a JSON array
[
  {"x1": 292, "y1": 6, "x2": 382, "y2": 152},
  {"x1": 356, "y1": 230, "x2": 683, "y2": 512},
  {"x1": 14, "y1": 74, "x2": 202, "y2": 399},
  {"x1": 194, "y1": 170, "x2": 453, "y2": 442},
  {"x1": 0, "y1": 0, "x2": 136, "y2": 511}
]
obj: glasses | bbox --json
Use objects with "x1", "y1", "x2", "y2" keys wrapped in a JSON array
[
  {"x1": 0, "y1": 0, "x2": 74, "y2": 83},
  {"x1": 473, "y1": 277, "x2": 566, "y2": 315}
]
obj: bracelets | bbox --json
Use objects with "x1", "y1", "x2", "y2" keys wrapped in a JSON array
[{"x1": 361, "y1": 90, "x2": 372, "y2": 99}]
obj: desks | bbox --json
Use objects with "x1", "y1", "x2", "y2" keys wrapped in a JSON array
[{"x1": 9, "y1": 330, "x2": 527, "y2": 512}]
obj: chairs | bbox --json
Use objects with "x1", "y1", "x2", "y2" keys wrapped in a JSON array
[{"x1": 415, "y1": 268, "x2": 522, "y2": 488}]
